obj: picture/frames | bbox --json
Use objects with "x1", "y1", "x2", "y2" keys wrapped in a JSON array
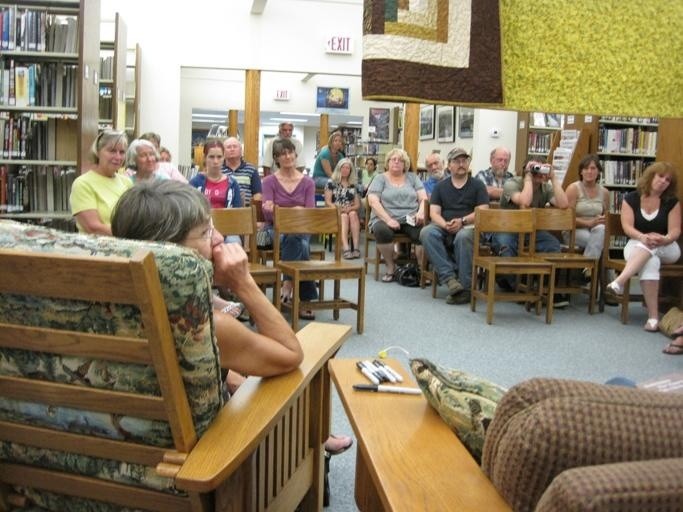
[
  {"x1": 458, "y1": 106, "x2": 473, "y2": 138},
  {"x1": 435, "y1": 105, "x2": 455, "y2": 143},
  {"x1": 419, "y1": 104, "x2": 435, "y2": 141}
]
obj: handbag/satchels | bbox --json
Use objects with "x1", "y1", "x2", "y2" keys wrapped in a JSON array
[
  {"x1": 255, "y1": 232, "x2": 271, "y2": 250},
  {"x1": 394, "y1": 267, "x2": 421, "y2": 287}
]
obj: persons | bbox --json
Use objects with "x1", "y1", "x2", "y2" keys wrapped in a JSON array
[
  {"x1": 311, "y1": 131, "x2": 682, "y2": 355},
  {"x1": 0, "y1": 4, "x2": 82, "y2": 235},
  {"x1": 108, "y1": 178, "x2": 355, "y2": 455},
  {"x1": 68, "y1": 123, "x2": 320, "y2": 322}
]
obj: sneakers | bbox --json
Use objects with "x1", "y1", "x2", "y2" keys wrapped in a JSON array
[
  {"x1": 553, "y1": 294, "x2": 569, "y2": 308},
  {"x1": 446, "y1": 278, "x2": 470, "y2": 304}
]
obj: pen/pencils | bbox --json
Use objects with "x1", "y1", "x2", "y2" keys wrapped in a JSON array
[{"x1": 352, "y1": 359, "x2": 421, "y2": 395}]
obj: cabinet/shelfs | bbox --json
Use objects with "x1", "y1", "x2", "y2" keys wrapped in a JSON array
[
  {"x1": 0, "y1": 0, "x2": 142, "y2": 232},
  {"x1": 516, "y1": 110, "x2": 683, "y2": 314}
]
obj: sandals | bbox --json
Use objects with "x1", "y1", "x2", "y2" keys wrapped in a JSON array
[
  {"x1": 325, "y1": 434, "x2": 353, "y2": 455},
  {"x1": 342, "y1": 247, "x2": 360, "y2": 259}
]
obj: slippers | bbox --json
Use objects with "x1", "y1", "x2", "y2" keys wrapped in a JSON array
[
  {"x1": 213, "y1": 300, "x2": 244, "y2": 318},
  {"x1": 606, "y1": 280, "x2": 624, "y2": 295},
  {"x1": 644, "y1": 317, "x2": 659, "y2": 331},
  {"x1": 300, "y1": 309, "x2": 315, "y2": 320},
  {"x1": 382, "y1": 272, "x2": 394, "y2": 282},
  {"x1": 662, "y1": 326, "x2": 683, "y2": 354},
  {"x1": 280, "y1": 285, "x2": 292, "y2": 304}
]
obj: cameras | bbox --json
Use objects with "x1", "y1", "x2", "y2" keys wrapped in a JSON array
[{"x1": 533, "y1": 164, "x2": 550, "y2": 174}]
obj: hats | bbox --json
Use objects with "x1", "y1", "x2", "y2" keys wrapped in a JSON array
[{"x1": 448, "y1": 146, "x2": 472, "y2": 159}]
